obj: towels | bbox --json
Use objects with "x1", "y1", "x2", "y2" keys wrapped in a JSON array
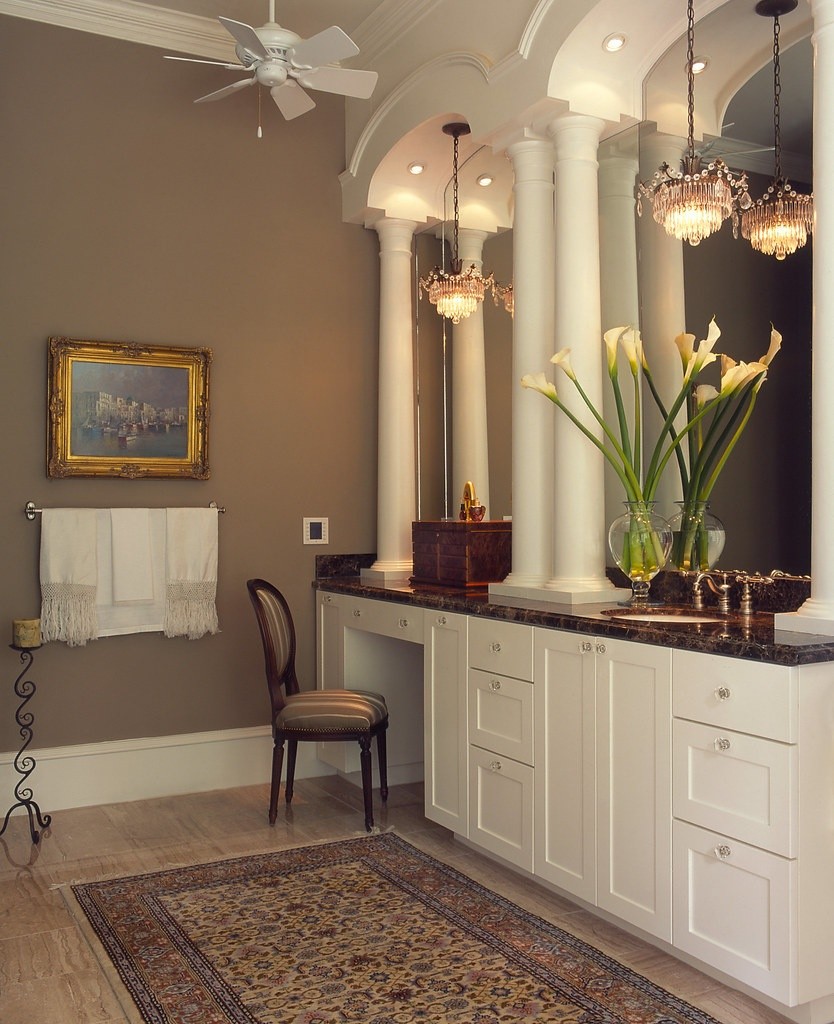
[{"x1": 39, "y1": 506, "x2": 217, "y2": 647}]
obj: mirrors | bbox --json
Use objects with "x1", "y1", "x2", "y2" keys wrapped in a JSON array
[
  {"x1": 598, "y1": 0, "x2": 814, "y2": 577},
  {"x1": 410, "y1": 143, "x2": 516, "y2": 520}
]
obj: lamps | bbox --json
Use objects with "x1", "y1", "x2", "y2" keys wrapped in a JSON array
[
  {"x1": 418, "y1": 123, "x2": 494, "y2": 325},
  {"x1": 741, "y1": 0, "x2": 816, "y2": 260},
  {"x1": 493, "y1": 281, "x2": 514, "y2": 317}
]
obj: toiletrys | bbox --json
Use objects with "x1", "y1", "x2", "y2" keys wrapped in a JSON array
[{"x1": 459, "y1": 481, "x2": 486, "y2": 521}]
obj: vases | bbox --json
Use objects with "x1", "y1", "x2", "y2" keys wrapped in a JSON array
[
  {"x1": 609, "y1": 499, "x2": 676, "y2": 611},
  {"x1": 671, "y1": 500, "x2": 728, "y2": 569}
]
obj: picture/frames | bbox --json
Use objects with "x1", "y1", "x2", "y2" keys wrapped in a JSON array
[{"x1": 46, "y1": 338, "x2": 213, "y2": 479}]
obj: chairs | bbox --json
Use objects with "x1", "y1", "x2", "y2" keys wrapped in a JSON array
[{"x1": 246, "y1": 578, "x2": 389, "y2": 832}]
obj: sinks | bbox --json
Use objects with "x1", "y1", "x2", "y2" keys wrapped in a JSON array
[{"x1": 600, "y1": 606, "x2": 724, "y2": 623}]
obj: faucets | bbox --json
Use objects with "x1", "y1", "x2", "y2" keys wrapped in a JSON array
[{"x1": 692, "y1": 572, "x2": 734, "y2": 615}]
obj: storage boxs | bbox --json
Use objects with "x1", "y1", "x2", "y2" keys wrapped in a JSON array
[{"x1": 409, "y1": 518, "x2": 512, "y2": 595}]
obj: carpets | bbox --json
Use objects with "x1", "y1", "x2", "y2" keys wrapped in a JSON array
[{"x1": 71, "y1": 831, "x2": 725, "y2": 1024}]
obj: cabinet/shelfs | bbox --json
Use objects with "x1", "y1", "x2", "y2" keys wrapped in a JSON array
[
  {"x1": 315, "y1": 596, "x2": 469, "y2": 840},
  {"x1": 531, "y1": 627, "x2": 676, "y2": 948},
  {"x1": 675, "y1": 648, "x2": 830, "y2": 1006},
  {"x1": 468, "y1": 618, "x2": 541, "y2": 874}
]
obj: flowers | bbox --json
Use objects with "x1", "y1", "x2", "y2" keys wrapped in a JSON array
[
  {"x1": 637, "y1": 319, "x2": 784, "y2": 499},
  {"x1": 521, "y1": 309, "x2": 757, "y2": 579}
]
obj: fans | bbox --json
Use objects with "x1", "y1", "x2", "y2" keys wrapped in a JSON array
[
  {"x1": 160, "y1": 0, "x2": 378, "y2": 138},
  {"x1": 637, "y1": 0, "x2": 752, "y2": 246}
]
